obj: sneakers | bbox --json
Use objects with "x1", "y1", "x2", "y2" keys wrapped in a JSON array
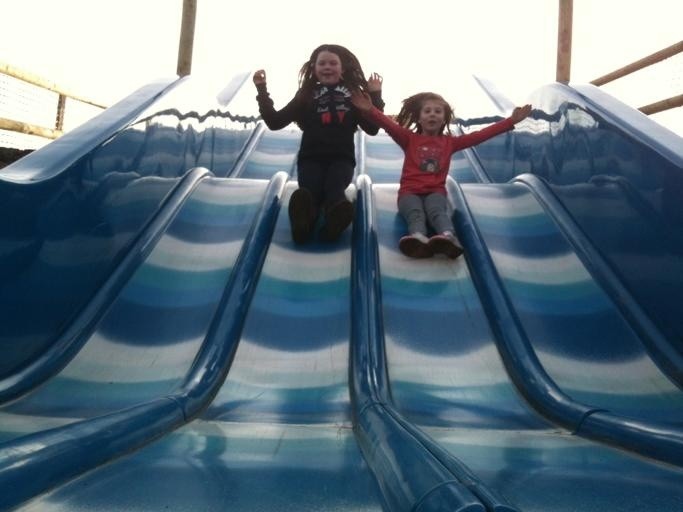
[
  {"x1": 289, "y1": 187, "x2": 354, "y2": 241},
  {"x1": 399, "y1": 232, "x2": 464, "y2": 259}
]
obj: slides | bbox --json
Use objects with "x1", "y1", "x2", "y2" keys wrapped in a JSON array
[{"x1": 0, "y1": 71, "x2": 683, "y2": 512}]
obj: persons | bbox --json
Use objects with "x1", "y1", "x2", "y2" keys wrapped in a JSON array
[
  {"x1": 251, "y1": 41, "x2": 388, "y2": 245},
  {"x1": 349, "y1": 79, "x2": 533, "y2": 262}
]
